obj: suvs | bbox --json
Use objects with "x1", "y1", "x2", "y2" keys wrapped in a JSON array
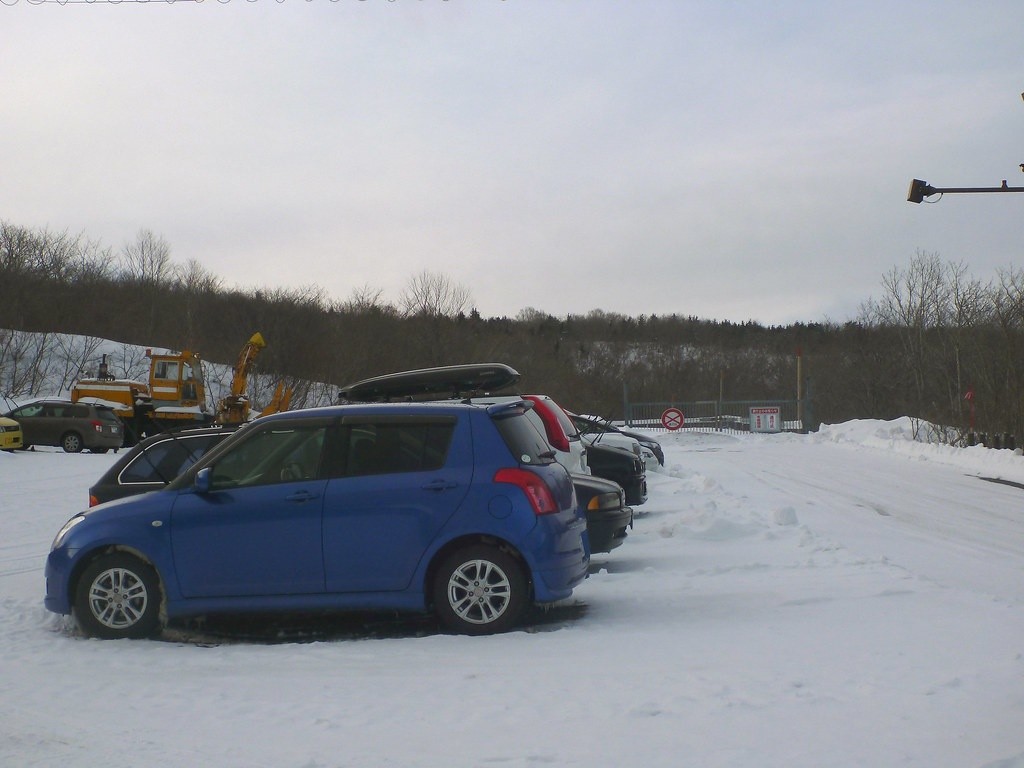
[{"x1": 2, "y1": 397, "x2": 125, "y2": 454}]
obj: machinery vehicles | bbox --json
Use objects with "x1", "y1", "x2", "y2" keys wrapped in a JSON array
[{"x1": 70, "y1": 330, "x2": 293, "y2": 448}]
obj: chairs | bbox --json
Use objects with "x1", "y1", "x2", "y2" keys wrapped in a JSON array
[{"x1": 350, "y1": 439, "x2": 379, "y2": 476}]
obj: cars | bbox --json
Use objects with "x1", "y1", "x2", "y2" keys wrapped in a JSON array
[
  {"x1": 334, "y1": 361, "x2": 665, "y2": 511},
  {"x1": 0, "y1": 414, "x2": 23, "y2": 452},
  {"x1": 43, "y1": 398, "x2": 591, "y2": 639},
  {"x1": 88, "y1": 420, "x2": 634, "y2": 555}
]
